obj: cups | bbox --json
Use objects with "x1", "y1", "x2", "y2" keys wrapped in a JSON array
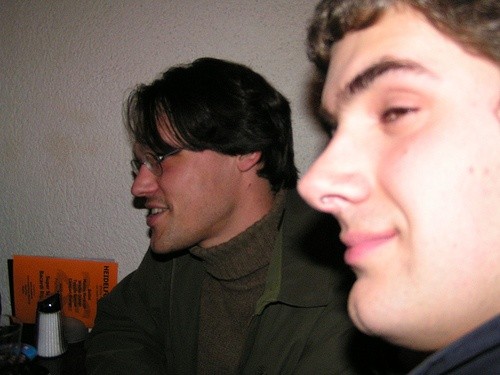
[{"x1": 0, "y1": 314, "x2": 23, "y2": 366}]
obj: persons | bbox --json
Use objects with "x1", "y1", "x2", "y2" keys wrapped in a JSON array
[
  {"x1": 294, "y1": 0, "x2": 500, "y2": 375},
  {"x1": 86, "y1": 58, "x2": 383, "y2": 375}
]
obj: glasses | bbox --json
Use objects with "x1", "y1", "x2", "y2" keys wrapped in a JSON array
[{"x1": 130, "y1": 144, "x2": 189, "y2": 178}]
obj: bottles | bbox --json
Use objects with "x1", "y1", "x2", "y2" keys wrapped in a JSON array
[{"x1": 32, "y1": 292, "x2": 75, "y2": 375}]
